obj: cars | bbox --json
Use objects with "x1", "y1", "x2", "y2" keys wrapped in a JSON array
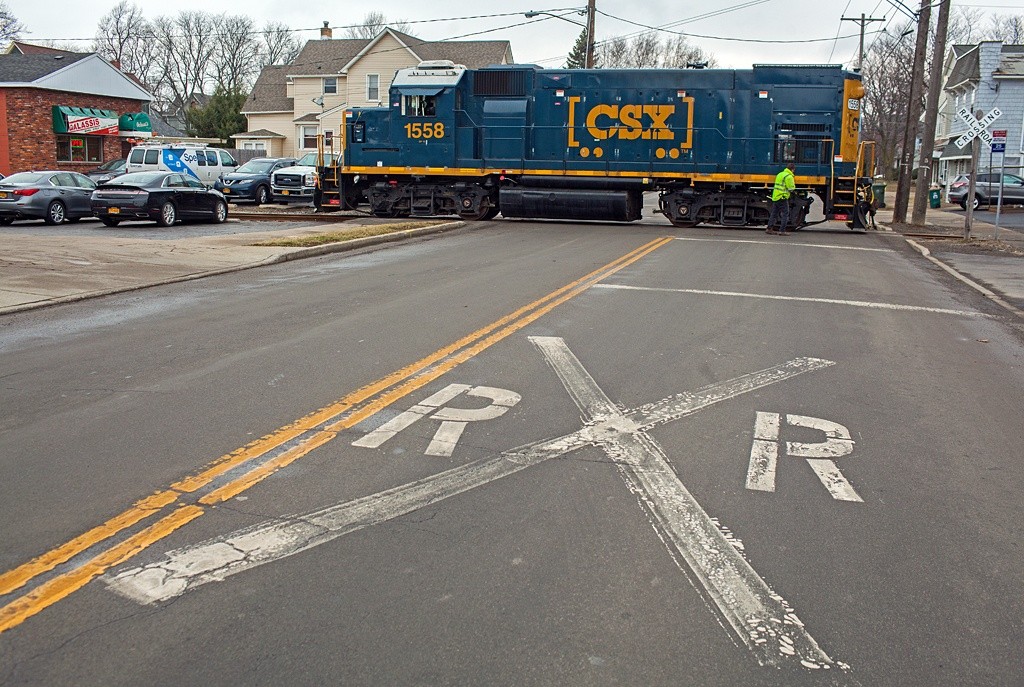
[
  {"x1": 0, "y1": 170, "x2": 99, "y2": 226},
  {"x1": 82, "y1": 158, "x2": 126, "y2": 185},
  {"x1": 90, "y1": 170, "x2": 229, "y2": 228}
]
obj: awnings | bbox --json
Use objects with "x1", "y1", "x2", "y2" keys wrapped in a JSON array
[
  {"x1": 119, "y1": 113, "x2": 152, "y2": 137},
  {"x1": 53, "y1": 106, "x2": 119, "y2": 136}
]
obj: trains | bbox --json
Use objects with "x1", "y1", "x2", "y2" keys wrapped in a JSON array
[{"x1": 340, "y1": 58, "x2": 878, "y2": 230}]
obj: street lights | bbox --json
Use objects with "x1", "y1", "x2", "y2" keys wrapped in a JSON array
[{"x1": 524, "y1": 11, "x2": 592, "y2": 69}]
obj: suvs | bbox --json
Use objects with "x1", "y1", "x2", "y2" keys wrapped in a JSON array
[
  {"x1": 947, "y1": 172, "x2": 1024, "y2": 211},
  {"x1": 213, "y1": 156, "x2": 300, "y2": 207},
  {"x1": 270, "y1": 151, "x2": 343, "y2": 206}
]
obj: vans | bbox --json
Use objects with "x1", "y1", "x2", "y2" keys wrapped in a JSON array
[{"x1": 125, "y1": 142, "x2": 240, "y2": 188}]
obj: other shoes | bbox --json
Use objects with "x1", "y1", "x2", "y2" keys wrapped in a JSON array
[
  {"x1": 777, "y1": 231, "x2": 790, "y2": 236},
  {"x1": 766, "y1": 229, "x2": 776, "y2": 234}
]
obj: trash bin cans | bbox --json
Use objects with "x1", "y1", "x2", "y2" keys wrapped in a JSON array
[
  {"x1": 872, "y1": 185, "x2": 888, "y2": 209},
  {"x1": 928, "y1": 189, "x2": 941, "y2": 209}
]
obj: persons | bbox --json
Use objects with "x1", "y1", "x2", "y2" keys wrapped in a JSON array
[{"x1": 765, "y1": 163, "x2": 795, "y2": 236}]
